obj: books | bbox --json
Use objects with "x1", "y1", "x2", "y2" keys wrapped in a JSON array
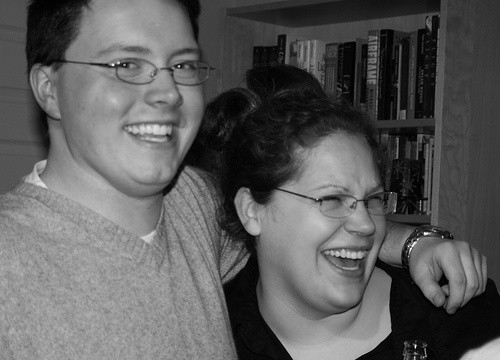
[
  {"x1": 373, "y1": 132, "x2": 435, "y2": 218},
  {"x1": 251, "y1": 15, "x2": 440, "y2": 120}
]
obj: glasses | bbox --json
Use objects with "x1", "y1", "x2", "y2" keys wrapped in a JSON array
[
  {"x1": 43, "y1": 57, "x2": 215, "y2": 85},
  {"x1": 263, "y1": 185, "x2": 398, "y2": 219}
]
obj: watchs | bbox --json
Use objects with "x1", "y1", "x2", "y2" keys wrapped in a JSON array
[{"x1": 401, "y1": 225, "x2": 455, "y2": 272}]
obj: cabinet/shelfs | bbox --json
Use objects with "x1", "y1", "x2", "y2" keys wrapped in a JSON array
[{"x1": 219, "y1": 0, "x2": 447, "y2": 228}]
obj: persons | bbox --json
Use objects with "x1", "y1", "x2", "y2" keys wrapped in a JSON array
[
  {"x1": 182, "y1": 86, "x2": 499, "y2": 359},
  {"x1": 1, "y1": 0, "x2": 486, "y2": 359}
]
